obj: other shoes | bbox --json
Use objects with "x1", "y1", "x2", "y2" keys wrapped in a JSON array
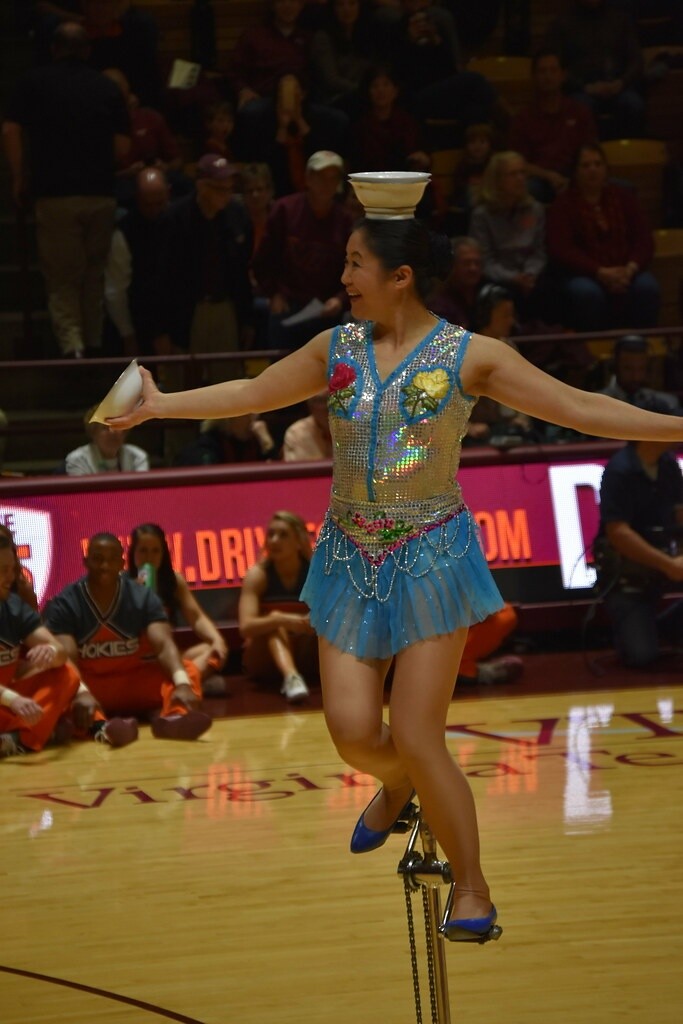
[
  {"x1": 203, "y1": 676, "x2": 226, "y2": 695},
  {"x1": 349, "y1": 779, "x2": 416, "y2": 852},
  {"x1": 56, "y1": 695, "x2": 94, "y2": 742},
  {"x1": 480, "y1": 655, "x2": 523, "y2": 684},
  {"x1": 260, "y1": 669, "x2": 279, "y2": 688},
  {"x1": 445, "y1": 902, "x2": 498, "y2": 939},
  {"x1": 94, "y1": 717, "x2": 138, "y2": 745},
  {"x1": 0, "y1": 734, "x2": 25, "y2": 760},
  {"x1": 150, "y1": 710, "x2": 212, "y2": 741},
  {"x1": 280, "y1": 673, "x2": 307, "y2": 702}
]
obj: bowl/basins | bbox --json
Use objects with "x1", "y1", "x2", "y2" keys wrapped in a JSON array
[
  {"x1": 348, "y1": 171, "x2": 432, "y2": 219},
  {"x1": 88, "y1": 357, "x2": 146, "y2": 426}
]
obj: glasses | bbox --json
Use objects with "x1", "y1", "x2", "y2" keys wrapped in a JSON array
[{"x1": 244, "y1": 187, "x2": 266, "y2": 197}]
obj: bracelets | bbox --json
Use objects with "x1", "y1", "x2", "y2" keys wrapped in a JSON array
[{"x1": 49, "y1": 644, "x2": 57, "y2": 661}]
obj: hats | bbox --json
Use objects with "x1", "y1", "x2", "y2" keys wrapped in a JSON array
[
  {"x1": 489, "y1": 152, "x2": 523, "y2": 174},
  {"x1": 194, "y1": 154, "x2": 238, "y2": 183},
  {"x1": 616, "y1": 335, "x2": 647, "y2": 354},
  {"x1": 307, "y1": 151, "x2": 344, "y2": 171}
]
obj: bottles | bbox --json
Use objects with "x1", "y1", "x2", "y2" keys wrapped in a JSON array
[
  {"x1": 138, "y1": 563, "x2": 155, "y2": 589},
  {"x1": 410, "y1": 8, "x2": 429, "y2": 45}
]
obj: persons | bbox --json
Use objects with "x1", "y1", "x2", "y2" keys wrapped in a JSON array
[
  {"x1": 39, "y1": 532, "x2": 212, "y2": 747},
  {"x1": 0, "y1": 0, "x2": 683, "y2": 479},
  {"x1": 105, "y1": 217, "x2": 683, "y2": 942},
  {"x1": 591, "y1": 388, "x2": 683, "y2": 671},
  {"x1": 0, "y1": 523, "x2": 81, "y2": 756},
  {"x1": 457, "y1": 602, "x2": 522, "y2": 688},
  {"x1": 127, "y1": 524, "x2": 228, "y2": 698},
  {"x1": 237, "y1": 511, "x2": 317, "y2": 702}
]
